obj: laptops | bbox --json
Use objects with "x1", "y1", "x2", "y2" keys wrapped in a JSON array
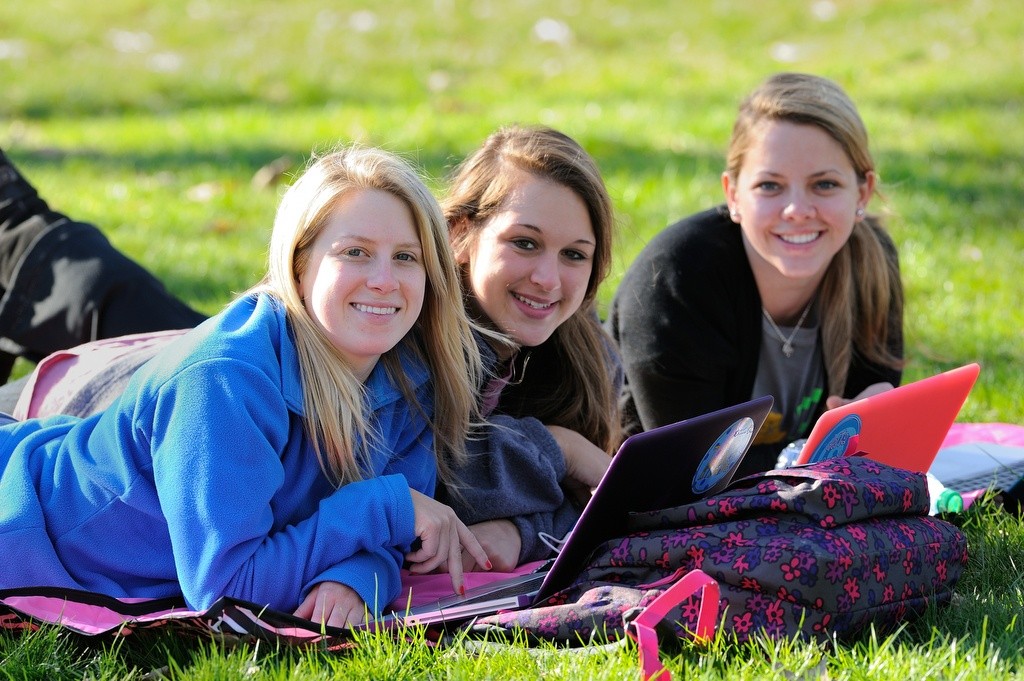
[
  {"x1": 353, "y1": 394, "x2": 774, "y2": 635},
  {"x1": 926, "y1": 440, "x2": 1024, "y2": 516}
]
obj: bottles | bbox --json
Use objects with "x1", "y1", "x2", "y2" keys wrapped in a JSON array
[{"x1": 775, "y1": 440, "x2": 964, "y2": 517}]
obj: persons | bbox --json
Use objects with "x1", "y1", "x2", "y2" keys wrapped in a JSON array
[
  {"x1": 0, "y1": 123, "x2": 645, "y2": 575},
  {"x1": 597, "y1": 73, "x2": 906, "y2": 482},
  {"x1": 0, "y1": 145, "x2": 492, "y2": 629}
]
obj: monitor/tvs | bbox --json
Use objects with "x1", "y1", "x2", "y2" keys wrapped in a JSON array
[{"x1": 793, "y1": 362, "x2": 982, "y2": 474}]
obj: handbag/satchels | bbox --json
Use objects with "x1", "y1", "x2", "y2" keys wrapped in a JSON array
[{"x1": 454, "y1": 450, "x2": 969, "y2": 650}]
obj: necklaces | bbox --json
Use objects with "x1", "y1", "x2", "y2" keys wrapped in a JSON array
[{"x1": 761, "y1": 297, "x2": 816, "y2": 357}]
